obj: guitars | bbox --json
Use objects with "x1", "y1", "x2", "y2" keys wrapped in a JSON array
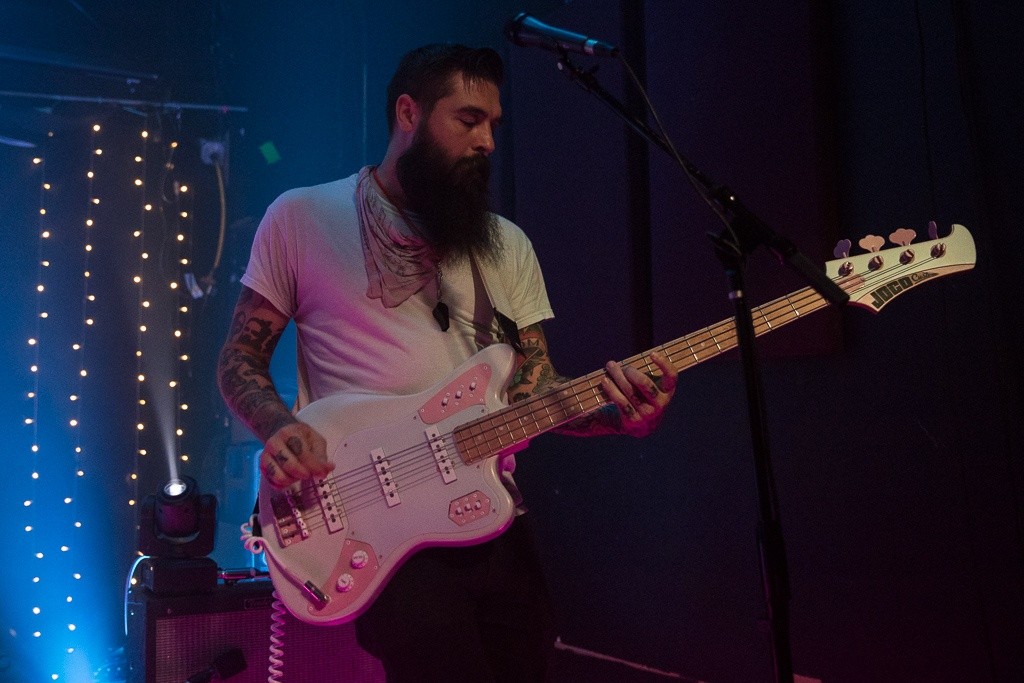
[{"x1": 255, "y1": 221, "x2": 977, "y2": 627}]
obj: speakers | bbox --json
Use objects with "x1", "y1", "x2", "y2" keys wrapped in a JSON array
[{"x1": 125, "y1": 581, "x2": 387, "y2": 683}]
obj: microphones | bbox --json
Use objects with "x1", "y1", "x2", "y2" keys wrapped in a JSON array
[
  {"x1": 184, "y1": 646, "x2": 247, "y2": 683},
  {"x1": 503, "y1": 10, "x2": 620, "y2": 59}
]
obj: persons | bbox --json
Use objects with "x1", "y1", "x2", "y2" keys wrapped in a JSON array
[{"x1": 215, "y1": 46, "x2": 681, "y2": 683}]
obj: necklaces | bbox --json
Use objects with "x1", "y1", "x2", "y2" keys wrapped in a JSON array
[{"x1": 372, "y1": 162, "x2": 450, "y2": 332}]
